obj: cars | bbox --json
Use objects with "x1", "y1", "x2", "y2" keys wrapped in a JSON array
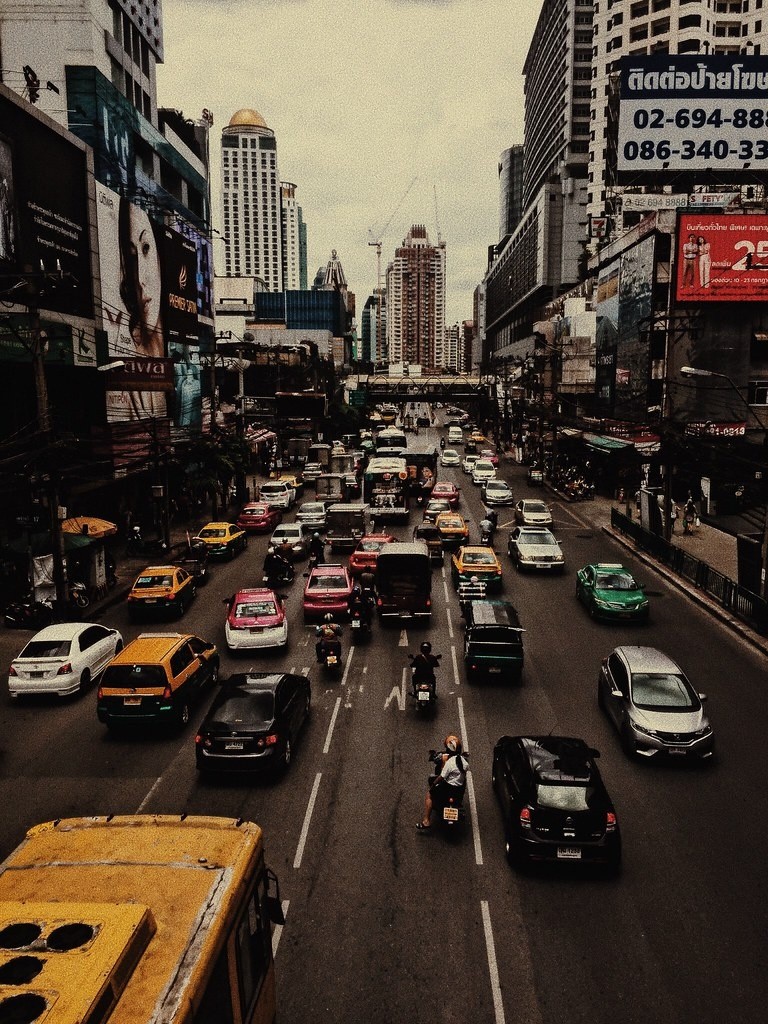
[
  {"x1": 597, "y1": 647, "x2": 714, "y2": 768},
  {"x1": 8, "y1": 623, "x2": 124, "y2": 700},
  {"x1": 196, "y1": 672, "x2": 311, "y2": 774},
  {"x1": 359, "y1": 403, "x2": 407, "y2": 447},
  {"x1": 491, "y1": 734, "x2": 622, "y2": 875},
  {"x1": 127, "y1": 463, "x2": 399, "y2": 651},
  {"x1": 346, "y1": 432, "x2": 373, "y2": 498},
  {"x1": 413, "y1": 406, "x2": 564, "y2": 685},
  {"x1": 576, "y1": 564, "x2": 649, "y2": 624}
]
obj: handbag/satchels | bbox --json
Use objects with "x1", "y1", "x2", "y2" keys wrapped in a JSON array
[
  {"x1": 696, "y1": 517, "x2": 701, "y2": 527},
  {"x1": 683, "y1": 520, "x2": 687, "y2": 527},
  {"x1": 675, "y1": 511, "x2": 679, "y2": 519}
]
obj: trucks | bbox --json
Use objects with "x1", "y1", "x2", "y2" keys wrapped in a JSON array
[{"x1": 308, "y1": 444, "x2": 353, "y2": 505}]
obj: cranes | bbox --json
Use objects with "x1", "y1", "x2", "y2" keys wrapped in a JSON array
[{"x1": 367, "y1": 176, "x2": 419, "y2": 286}]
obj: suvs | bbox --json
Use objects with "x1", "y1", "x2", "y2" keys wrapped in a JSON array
[{"x1": 96, "y1": 632, "x2": 220, "y2": 732}]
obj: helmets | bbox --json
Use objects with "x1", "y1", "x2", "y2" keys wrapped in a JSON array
[
  {"x1": 282, "y1": 537, "x2": 288, "y2": 542},
  {"x1": 365, "y1": 565, "x2": 371, "y2": 571},
  {"x1": 444, "y1": 735, "x2": 462, "y2": 751},
  {"x1": 134, "y1": 526, "x2": 140, "y2": 533},
  {"x1": 313, "y1": 532, "x2": 319, "y2": 538},
  {"x1": 420, "y1": 641, "x2": 432, "y2": 653},
  {"x1": 470, "y1": 576, "x2": 478, "y2": 582},
  {"x1": 324, "y1": 613, "x2": 334, "y2": 622},
  {"x1": 267, "y1": 547, "x2": 274, "y2": 554},
  {"x1": 352, "y1": 586, "x2": 360, "y2": 595}
]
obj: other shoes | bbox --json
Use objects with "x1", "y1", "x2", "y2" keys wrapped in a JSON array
[
  {"x1": 432, "y1": 694, "x2": 438, "y2": 699},
  {"x1": 338, "y1": 659, "x2": 342, "y2": 665},
  {"x1": 316, "y1": 660, "x2": 326, "y2": 664},
  {"x1": 408, "y1": 692, "x2": 417, "y2": 698}
]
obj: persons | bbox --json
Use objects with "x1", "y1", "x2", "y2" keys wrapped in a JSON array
[
  {"x1": 263, "y1": 539, "x2": 295, "y2": 578},
  {"x1": 479, "y1": 516, "x2": 495, "y2": 547},
  {"x1": 410, "y1": 642, "x2": 439, "y2": 699},
  {"x1": 680, "y1": 233, "x2": 711, "y2": 288},
  {"x1": 120, "y1": 197, "x2": 166, "y2": 356},
  {"x1": 349, "y1": 566, "x2": 378, "y2": 634},
  {"x1": 316, "y1": 613, "x2": 343, "y2": 664},
  {"x1": 441, "y1": 436, "x2": 445, "y2": 444},
  {"x1": 309, "y1": 533, "x2": 326, "y2": 564},
  {"x1": 671, "y1": 498, "x2": 698, "y2": 535},
  {"x1": 416, "y1": 735, "x2": 469, "y2": 829}
]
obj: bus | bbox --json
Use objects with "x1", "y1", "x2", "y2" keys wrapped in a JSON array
[
  {"x1": 374, "y1": 542, "x2": 432, "y2": 627},
  {"x1": 324, "y1": 504, "x2": 374, "y2": 552},
  {"x1": 364, "y1": 431, "x2": 439, "y2": 517},
  {"x1": 0, "y1": 812, "x2": 286, "y2": 1024}
]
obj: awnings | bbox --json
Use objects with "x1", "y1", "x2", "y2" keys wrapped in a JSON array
[{"x1": 561, "y1": 428, "x2": 630, "y2": 454}]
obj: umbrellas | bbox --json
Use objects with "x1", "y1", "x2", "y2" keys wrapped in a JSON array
[{"x1": 60, "y1": 516, "x2": 117, "y2": 538}]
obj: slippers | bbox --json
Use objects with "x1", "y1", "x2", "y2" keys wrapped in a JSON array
[{"x1": 415, "y1": 822, "x2": 432, "y2": 829}]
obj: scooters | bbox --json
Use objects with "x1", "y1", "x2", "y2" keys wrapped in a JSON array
[
  {"x1": 316, "y1": 625, "x2": 342, "y2": 671},
  {"x1": 4, "y1": 576, "x2": 89, "y2": 630},
  {"x1": 428, "y1": 750, "x2": 470, "y2": 826},
  {"x1": 407, "y1": 654, "x2": 442, "y2": 711},
  {"x1": 350, "y1": 588, "x2": 374, "y2": 636}
]
